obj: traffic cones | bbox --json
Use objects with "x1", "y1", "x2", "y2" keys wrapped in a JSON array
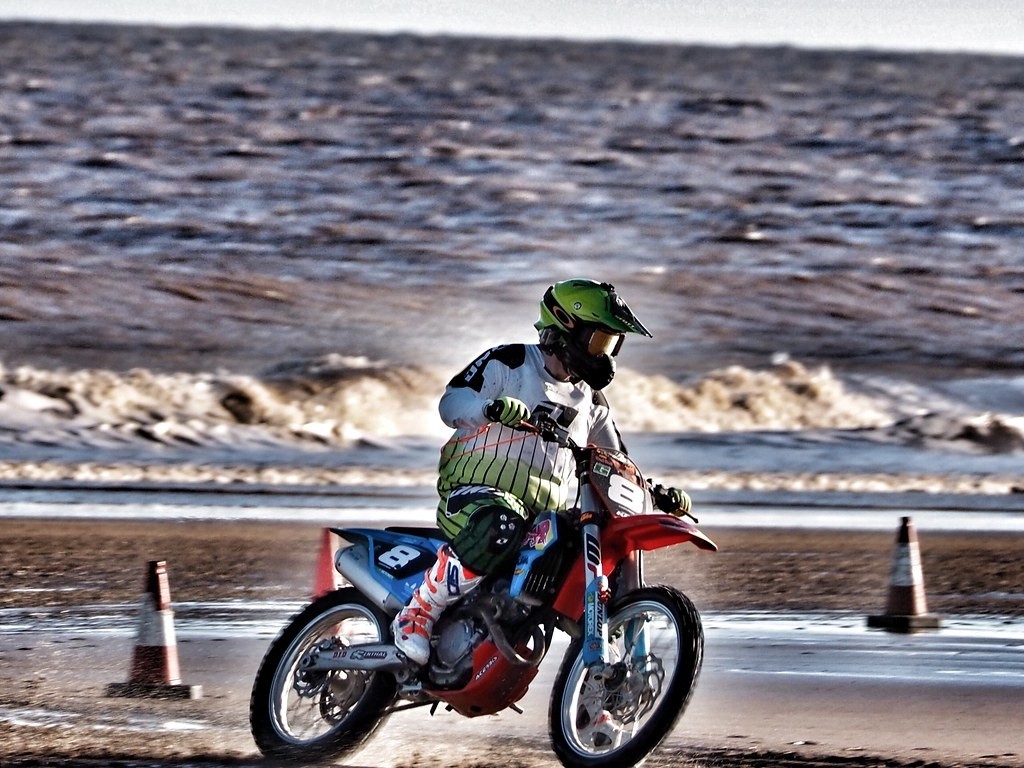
[
  {"x1": 104, "y1": 559, "x2": 205, "y2": 701},
  {"x1": 865, "y1": 518, "x2": 940, "y2": 631},
  {"x1": 311, "y1": 527, "x2": 345, "y2": 639}
]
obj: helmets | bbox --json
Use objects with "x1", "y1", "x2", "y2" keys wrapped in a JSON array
[{"x1": 533, "y1": 279, "x2": 653, "y2": 391}]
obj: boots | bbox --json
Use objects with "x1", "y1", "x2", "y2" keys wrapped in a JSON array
[{"x1": 389, "y1": 540, "x2": 488, "y2": 666}]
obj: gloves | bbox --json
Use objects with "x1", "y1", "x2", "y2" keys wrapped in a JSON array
[
  {"x1": 647, "y1": 478, "x2": 692, "y2": 517},
  {"x1": 482, "y1": 396, "x2": 531, "y2": 430}
]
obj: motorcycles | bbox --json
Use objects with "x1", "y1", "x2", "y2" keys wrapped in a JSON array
[{"x1": 249, "y1": 407, "x2": 720, "y2": 768}]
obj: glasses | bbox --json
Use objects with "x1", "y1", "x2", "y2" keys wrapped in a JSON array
[{"x1": 580, "y1": 322, "x2": 626, "y2": 357}]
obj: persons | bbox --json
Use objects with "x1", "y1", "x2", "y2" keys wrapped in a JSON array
[{"x1": 391, "y1": 278, "x2": 691, "y2": 666}]
